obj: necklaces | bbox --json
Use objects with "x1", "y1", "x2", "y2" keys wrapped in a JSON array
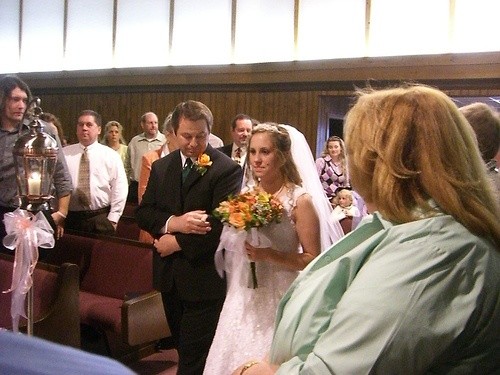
[{"x1": 256, "y1": 177, "x2": 285, "y2": 200}]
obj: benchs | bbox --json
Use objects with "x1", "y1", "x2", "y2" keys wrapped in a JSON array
[{"x1": 0, "y1": 203, "x2": 173, "y2": 366}]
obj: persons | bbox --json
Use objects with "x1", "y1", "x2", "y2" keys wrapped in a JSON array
[
  {"x1": 315, "y1": 136, "x2": 350, "y2": 210},
  {"x1": 138, "y1": 112, "x2": 179, "y2": 244},
  {"x1": 0, "y1": 74, "x2": 73, "y2": 264},
  {"x1": 134, "y1": 100, "x2": 243, "y2": 375},
  {"x1": 203, "y1": 122, "x2": 345, "y2": 374},
  {"x1": 232, "y1": 76, "x2": 500, "y2": 375},
  {"x1": 99, "y1": 121, "x2": 131, "y2": 187},
  {"x1": 127, "y1": 112, "x2": 167, "y2": 206},
  {"x1": 62, "y1": 110, "x2": 128, "y2": 236},
  {"x1": 332, "y1": 188, "x2": 360, "y2": 235},
  {"x1": 215, "y1": 113, "x2": 253, "y2": 167}
]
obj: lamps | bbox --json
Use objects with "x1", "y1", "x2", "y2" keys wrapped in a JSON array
[{"x1": 10, "y1": 95, "x2": 60, "y2": 337}]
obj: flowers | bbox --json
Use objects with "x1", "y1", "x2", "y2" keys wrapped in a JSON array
[
  {"x1": 213, "y1": 186, "x2": 289, "y2": 290},
  {"x1": 191, "y1": 153, "x2": 213, "y2": 176}
]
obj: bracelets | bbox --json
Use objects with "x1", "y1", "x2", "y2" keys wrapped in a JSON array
[
  {"x1": 240, "y1": 361, "x2": 261, "y2": 375},
  {"x1": 58, "y1": 211, "x2": 66, "y2": 219}
]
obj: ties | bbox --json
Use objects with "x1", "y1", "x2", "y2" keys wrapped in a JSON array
[
  {"x1": 76, "y1": 148, "x2": 91, "y2": 206},
  {"x1": 235, "y1": 148, "x2": 242, "y2": 163},
  {"x1": 181, "y1": 158, "x2": 193, "y2": 186}
]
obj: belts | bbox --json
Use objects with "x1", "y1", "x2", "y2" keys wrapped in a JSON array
[{"x1": 68, "y1": 206, "x2": 110, "y2": 219}]
